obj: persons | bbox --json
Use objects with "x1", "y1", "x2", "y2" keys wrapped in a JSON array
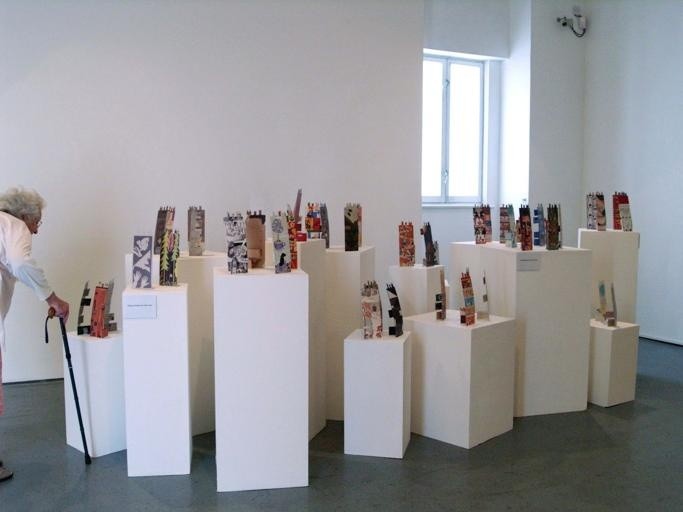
[{"x1": 0, "y1": 184, "x2": 70, "y2": 482}]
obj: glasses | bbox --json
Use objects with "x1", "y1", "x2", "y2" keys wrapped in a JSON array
[{"x1": 37, "y1": 220, "x2": 42, "y2": 226}]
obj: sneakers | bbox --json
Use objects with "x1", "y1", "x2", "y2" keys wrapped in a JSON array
[{"x1": 0, "y1": 461, "x2": 13, "y2": 482}]
